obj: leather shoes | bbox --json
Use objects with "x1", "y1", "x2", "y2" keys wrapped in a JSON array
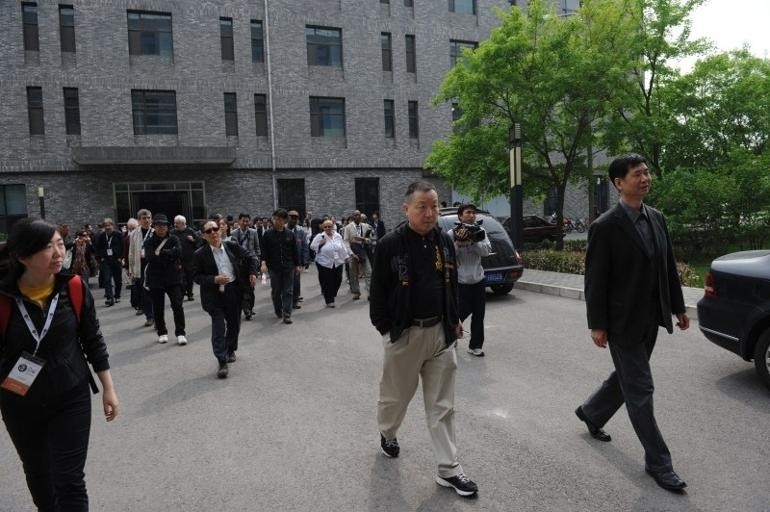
[
  {"x1": 645, "y1": 462, "x2": 687, "y2": 491},
  {"x1": 104, "y1": 298, "x2": 114, "y2": 305},
  {"x1": 327, "y1": 303, "x2": 335, "y2": 308},
  {"x1": 136, "y1": 308, "x2": 157, "y2": 330},
  {"x1": 574, "y1": 405, "x2": 612, "y2": 442},
  {"x1": 277, "y1": 294, "x2": 303, "y2": 324}
]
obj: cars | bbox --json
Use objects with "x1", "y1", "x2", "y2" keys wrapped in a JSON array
[
  {"x1": 493, "y1": 215, "x2": 566, "y2": 245},
  {"x1": 697, "y1": 249, "x2": 770, "y2": 389}
]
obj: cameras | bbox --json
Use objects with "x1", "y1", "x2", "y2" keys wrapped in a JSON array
[{"x1": 322, "y1": 235, "x2": 324, "y2": 239}]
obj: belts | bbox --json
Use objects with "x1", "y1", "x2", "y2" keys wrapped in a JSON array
[{"x1": 414, "y1": 319, "x2": 441, "y2": 328}]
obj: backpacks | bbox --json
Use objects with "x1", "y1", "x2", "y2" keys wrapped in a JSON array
[{"x1": 309, "y1": 232, "x2": 325, "y2": 261}]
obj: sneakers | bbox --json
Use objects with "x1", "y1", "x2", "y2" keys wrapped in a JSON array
[
  {"x1": 217, "y1": 364, "x2": 229, "y2": 378},
  {"x1": 436, "y1": 474, "x2": 477, "y2": 497},
  {"x1": 177, "y1": 335, "x2": 187, "y2": 345},
  {"x1": 468, "y1": 348, "x2": 485, "y2": 357},
  {"x1": 227, "y1": 350, "x2": 237, "y2": 362},
  {"x1": 158, "y1": 335, "x2": 168, "y2": 343},
  {"x1": 380, "y1": 434, "x2": 400, "y2": 458}
]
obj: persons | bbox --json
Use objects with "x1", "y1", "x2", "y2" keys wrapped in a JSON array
[
  {"x1": 59, "y1": 218, "x2": 139, "y2": 305},
  {"x1": 368, "y1": 182, "x2": 478, "y2": 498},
  {"x1": 574, "y1": 152, "x2": 690, "y2": 491},
  {"x1": 189, "y1": 212, "x2": 273, "y2": 320},
  {"x1": 187, "y1": 219, "x2": 257, "y2": 379},
  {"x1": 446, "y1": 204, "x2": 493, "y2": 356},
  {"x1": 0, "y1": 217, "x2": 120, "y2": 510},
  {"x1": 261, "y1": 210, "x2": 384, "y2": 324},
  {"x1": 129, "y1": 209, "x2": 157, "y2": 327},
  {"x1": 141, "y1": 211, "x2": 189, "y2": 345}
]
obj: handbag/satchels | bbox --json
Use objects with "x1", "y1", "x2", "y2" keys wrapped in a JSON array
[{"x1": 61, "y1": 249, "x2": 73, "y2": 270}]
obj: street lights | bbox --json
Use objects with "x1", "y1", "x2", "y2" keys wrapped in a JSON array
[
  {"x1": 597, "y1": 175, "x2": 602, "y2": 215},
  {"x1": 38, "y1": 186, "x2": 45, "y2": 220},
  {"x1": 508, "y1": 123, "x2": 523, "y2": 253}
]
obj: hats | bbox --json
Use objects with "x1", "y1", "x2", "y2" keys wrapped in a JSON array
[
  {"x1": 150, "y1": 214, "x2": 171, "y2": 227},
  {"x1": 288, "y1": 211, "x2": 299, "y2": 216}
]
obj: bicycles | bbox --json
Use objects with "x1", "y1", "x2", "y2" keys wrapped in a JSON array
[{"x1": 542, "y1": 216, "x2": 588, "y2": 234}]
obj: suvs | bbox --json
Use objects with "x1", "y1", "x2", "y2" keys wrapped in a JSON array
[{"x1": 394, "y1": 208, "x2": 523, "y2": 296}]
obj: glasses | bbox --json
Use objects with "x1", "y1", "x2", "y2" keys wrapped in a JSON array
[{"x1": 204, "y1": 227, "x2": 219, "y2": 233}]
